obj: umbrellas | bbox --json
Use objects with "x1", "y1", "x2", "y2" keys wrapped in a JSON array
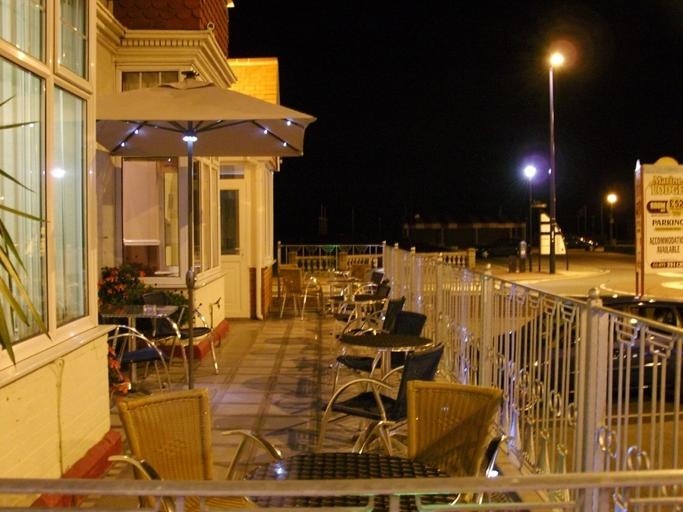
[{"x1": 94, "y1": 68, "x2": 319, "y2": 391}]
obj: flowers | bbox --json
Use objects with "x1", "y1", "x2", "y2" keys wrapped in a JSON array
[{"x1": 96, "y1": 262, "x2": 144, "y2": 306}]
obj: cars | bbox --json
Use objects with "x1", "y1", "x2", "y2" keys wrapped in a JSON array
[
  {"x1": 457, "y1": 294, "x2": 683, "y2": 412},
  {"x1": 477, "y1": 237, "x2": 533, "y2": 259}
]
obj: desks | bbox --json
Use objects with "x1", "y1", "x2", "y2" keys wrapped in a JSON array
[{"x1": 99, "y1": 304, "x2": 179, "y2": 393}]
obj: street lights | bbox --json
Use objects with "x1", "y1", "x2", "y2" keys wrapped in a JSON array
[
  {"x1": 549, "y1": 51, "x2": 564, "y2": 274},
  {"x1": 608, "y1": 194, "x2": 617, "y2": 242},
  {"x1": 525, "y1": 165, "x2": 535, "y2": 272}
]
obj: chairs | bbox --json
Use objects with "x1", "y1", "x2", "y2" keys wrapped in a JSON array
[
  {"x1": 108, "y1": 386, "x2": 285, "y2": 512},
  {"x1": 279, "y1": 263, "x2": 509, "y2": 512},
  {"x1": 108, "y1": 324, "x2": 173, "y2": 391},
  {"x1": 143, "y1": 289, "x2": 219, "y2": 384}
]
